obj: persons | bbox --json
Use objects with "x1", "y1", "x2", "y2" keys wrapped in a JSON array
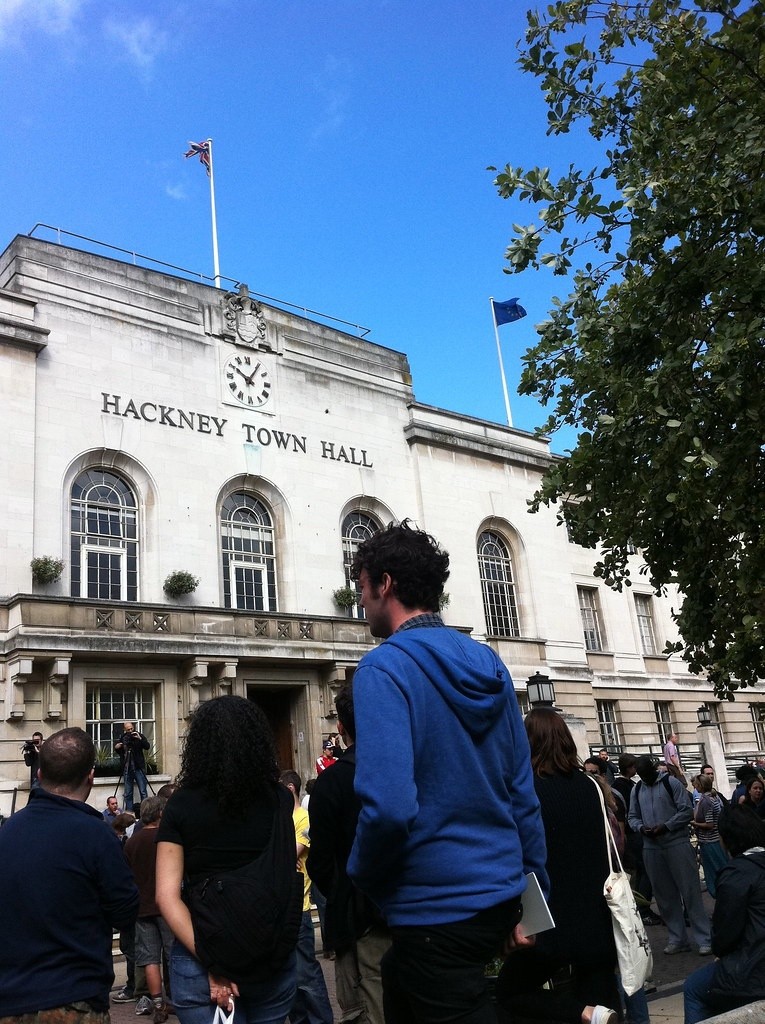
[
  {"x1": 306, "y1": 681, "x2": 765, "y2": 1024},
  {"x1": 156, "y1": 694, "x2": 305, "y2": 1024},
  {"x1": 24, "y1": 732, "x2": 43, "y2": 784},
  {"x1": 114, "y1": 722, "x2": 150, "y2": 811},
  {"x1": 104, "y1": 768, "x2": 336, "y2": 1024},
  {"x1": 345, "y1": 519, "x2": 550, "y2": 1024},
  {"x1": 0, "y1": 726, "x2": 140, "y2": 1024}
]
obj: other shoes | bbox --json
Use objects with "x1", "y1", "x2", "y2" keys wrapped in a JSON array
[
  {"x1": 323, "y1": 949, "x2": 337, "y2": 961},
  {"x1": 590, "y1": 1003, "x2": 618, "y2": 1024},
  {"x1": 154, "y1": 996, "x2": 164, "y2": 1009},
  {"x1": 642, "y1": 916, "x2": 660, "y2": 925},
  {"x1": 698, "y1": 944, "x2": 712, "y2": 954},
  {"x1": 663, "y1": 941, "x2": 690, "y2": 955}
]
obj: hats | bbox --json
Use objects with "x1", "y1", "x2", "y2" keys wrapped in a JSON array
[{"x1": 323, "y1": 740, "x2": 335, "y2": 749}]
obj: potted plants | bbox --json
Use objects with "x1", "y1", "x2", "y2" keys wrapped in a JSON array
[{"x1": 93, "y1": 740, "x2": 163, "y2": 777}]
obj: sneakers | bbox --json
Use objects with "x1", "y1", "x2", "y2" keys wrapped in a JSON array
[
  {"x1": 134, "y1": 996, "x2": 154, "y2": 1015},
  {"x1": 112, "y1": 991, "x2": 139, "y2": 1003}
]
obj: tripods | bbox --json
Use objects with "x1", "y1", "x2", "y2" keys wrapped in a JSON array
[{"x1": 113, "y1": 745, "x2": 156, "y2": 810}]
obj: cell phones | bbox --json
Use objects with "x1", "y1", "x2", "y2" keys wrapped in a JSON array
[{"x1": 646, "y1": 830, "x2": 652, "y2": 832}]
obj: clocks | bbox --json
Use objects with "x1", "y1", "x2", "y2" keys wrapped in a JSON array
[{"x1": 224, "y1": 351, "x2": 274, "y2": 409}]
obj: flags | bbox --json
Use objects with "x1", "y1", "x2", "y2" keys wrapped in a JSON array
[
  {"x1": 493, "y1": 297, "x2": 527, "y2": 326},
  {"x1": 183, "y1": 140, "x2": 211, "y2": 175}
]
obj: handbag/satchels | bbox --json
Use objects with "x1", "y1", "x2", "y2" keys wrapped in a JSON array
[
  {"x1": 584, "y1": 773, "x2": 656, "y2": 996},
  {"x1": 188, "y1": 875, "x2": 280, "y2": 976}
]
